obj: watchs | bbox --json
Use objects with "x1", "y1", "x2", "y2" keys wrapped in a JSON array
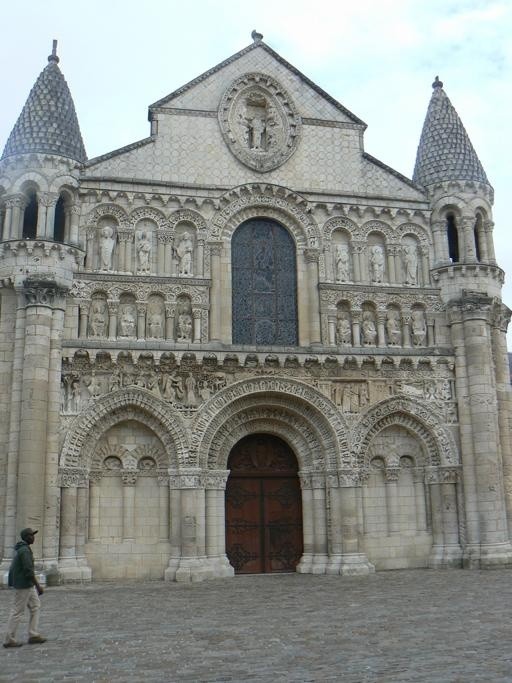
[{"x1": 71, "y1": 367, "x2": 213, "y2": 411}]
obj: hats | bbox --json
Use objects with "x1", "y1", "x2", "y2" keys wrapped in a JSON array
[{"x1": 20, "y1": 528, "x2": 38, "y2": 540}]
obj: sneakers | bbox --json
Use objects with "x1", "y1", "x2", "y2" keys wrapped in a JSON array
[
  {"x1": 28, "y1": 636, "x2": 47, "y2": 644},
  {"x1": 3, "y1": 642, "x2": 23, "y2": 648}
]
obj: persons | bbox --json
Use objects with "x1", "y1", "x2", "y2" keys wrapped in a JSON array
[
  {"x1": 410, "y1": 307, "x2": 426, "y2": 345},
  {"x1": 331, "y1": 382, "x2": 368, "y2": 412},
  {"x1": 2, "y1": 528, "x2": 46, "y2": 650},
  {"x1": 89, "y1": 302, "x2": 106, "y2": 337},
  {"x1": 338, "y1": 312, "x2": 352, "y2": 341},
  {"x1": 136, "y1": 231, "x2": 152, "y2": 274},
  {"x1": 251, "y1": 117, "x2": 264, "y2": 147},
  {"x1": 368, "y1": 247, "x2": 386, "y2": 285},
  {"x1": 99, "y1": 227, "x2": 114, "y2": 273},
  {"x1": 387, "y1": 311, "x2": 401, "y2": 341},
  {"x1": 181, "y1": 306, "x2": 196, "y2": 341},
  {"x1": 364, "y1": 315, "x2": 378, "y2": 344},
  {"x1": 173, "y1": 231, "x2": 193, "y2": 277},
  {"x1": 404, "y1": 247, "x2": 419, "y2": 286},
  {"x1": 336, "y1": 245, "x2": 350, "y2": 284},
  {"x1": 120, "y1": 306, "x2": 137, "y2": 341}
]
